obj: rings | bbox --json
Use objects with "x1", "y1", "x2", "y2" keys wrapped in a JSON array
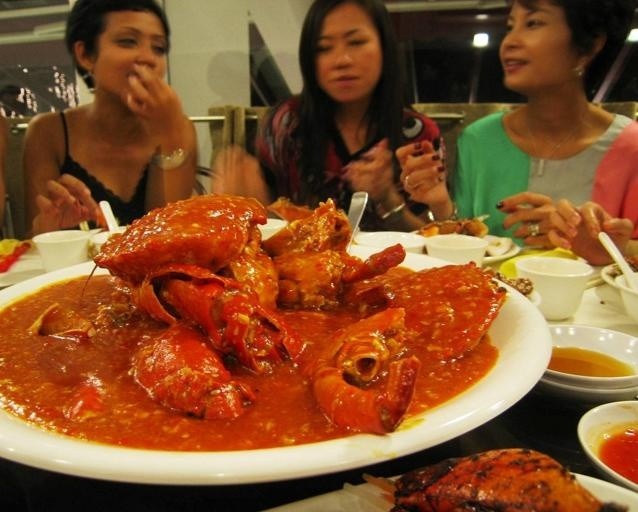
[
  {"x1": 404, "y1": 176, "x2": 408, "y2": 192},
  {"x1": 528, "y1": 224, "x2": 541, "y2": 239}
]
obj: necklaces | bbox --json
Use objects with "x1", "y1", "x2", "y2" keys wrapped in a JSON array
[{"x1": 518, "y1": 104, "x2": 601, "y2": 176}]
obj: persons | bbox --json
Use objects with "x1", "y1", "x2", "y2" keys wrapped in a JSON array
[
  {"x1": 22, "y1": 0, "x2": 198, "y2": 239},
  {"x1": 0, "y1": 85, "x2": 35, "y2": 118},
  {"x1": 395, "y1": 0, "x2": 638, "y2": 249},
  {"x1": 210, "y1": 0, "x2": 446, "y2": 232},
  {"x1": 548, "y1": 122, "x2": 638, "y2": 266},
  {"x1": 0, "y1": 117, "x2": 9, "y2": 229}
]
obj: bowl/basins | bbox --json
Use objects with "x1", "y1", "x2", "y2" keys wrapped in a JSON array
[
  {"x1": 601, "y1": 263, "x2": 636, "y2": 290},
  {"x1": 541, "y1": 325, "x2": 638, "y2": 402},
  {"x1": 423, "y1": 233, "x2": 488, "y2": 268},
  {"x1": 91, "y1": 226, "x2": 130, "y2": 253},
  {"x1": 577, "y1": 400, "x2": 638, "y2": 493},
  {"x1": 358, "y1": 229, "x2": 426, "y2": 255},
  {"x1": 509, "y1": 253, "x2": 595, "y2": 322},
  {"x1": 30, "y1": 229, "x2": 87, "y2": 272},
  {"x1": 613, "y1": 271, "x2": 638, "y2": 322}
]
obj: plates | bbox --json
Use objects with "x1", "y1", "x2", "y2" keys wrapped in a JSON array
[
  {"x1": 263, "y1": 470, "x2": 638, "y2": 512},
  {"x1": 498, "y1": 251, "x2": 588, "y2": 280},
  {"x1": 3, "y1": 244, "x2": 554, "y2": 486},
  {"x1": 476, "y1": 236, "x2": 521, "y2": 263}
]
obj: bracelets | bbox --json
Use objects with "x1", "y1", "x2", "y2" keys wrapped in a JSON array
[
  {"x1": 382, "y1": 202, "x2": 406, "y2": 219},
  {"x1": 157, "y1": 148, "x2": 190, "y2": 170}
]
